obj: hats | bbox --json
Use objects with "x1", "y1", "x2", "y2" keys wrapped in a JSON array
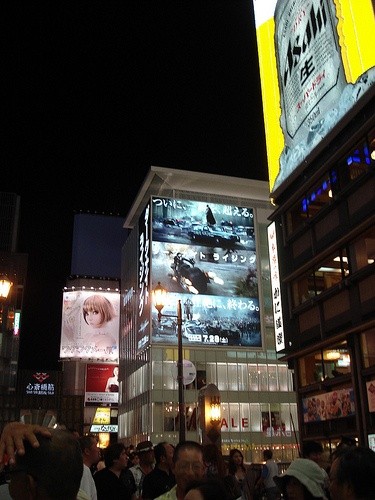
[
  {"x1": 132, "y1": 441, "x2": 154, "y2": 455},
  {"x1": 273, "y1": 458, "x2": 329, "y2": 500}
]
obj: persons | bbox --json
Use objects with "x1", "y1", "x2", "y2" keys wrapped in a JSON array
[
  {"x1": 157, "y1": 203, "x2": 259, "y2": 344},
  {"x1": 0, "y1": 421, "x2": 375, "y2": 500},
  {"x1": 105, "y1": 366, "x2": 120, "y2": 393},
  {"x1": 64, "y1": 295, "x2": 116, "y2": 347}
]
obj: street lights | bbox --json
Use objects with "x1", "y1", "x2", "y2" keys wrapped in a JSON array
[
  {"x1": 154, "y1": 281, "x2": 187, "y2": 446},
  {"x1": 0, "y1": 274, "x2": 14, "y2": 399}
]
